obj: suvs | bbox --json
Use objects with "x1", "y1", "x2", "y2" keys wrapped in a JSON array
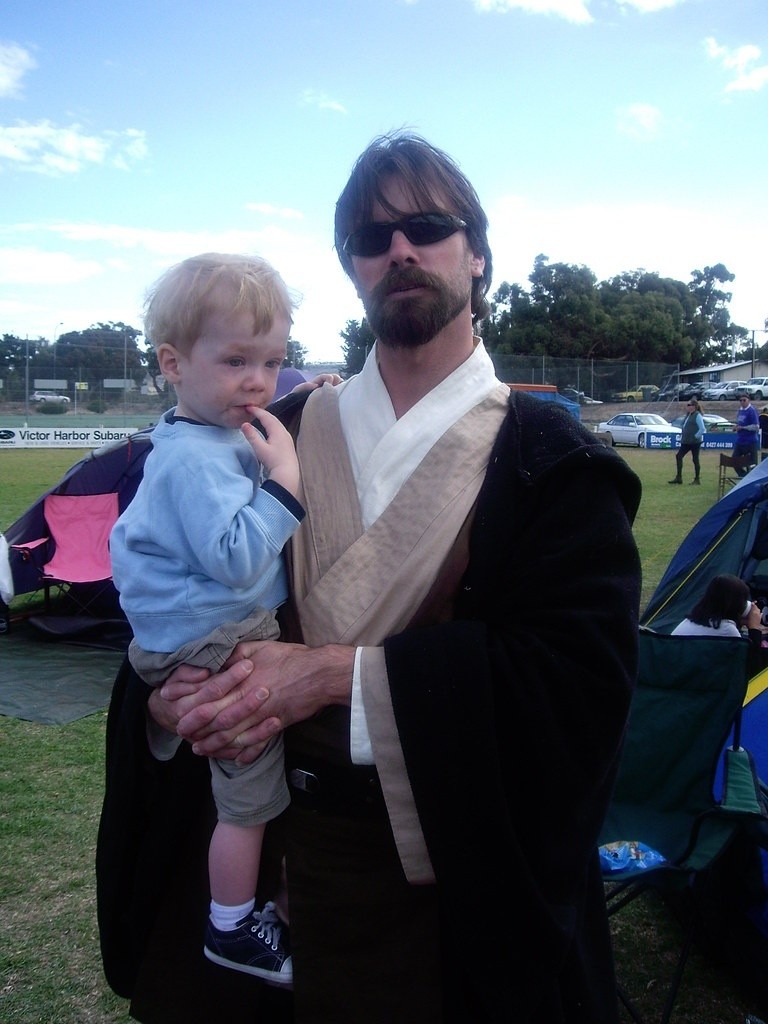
[{"x1": 733, "y1": 377, "x2": 768, "y2": 401}]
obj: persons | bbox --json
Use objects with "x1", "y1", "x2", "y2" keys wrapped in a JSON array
[
  {"x1": 730, "y1": 393, "x2": 759, "y2": 479},
  {"x1": 97, "y1": 136, "x2": 642, "y2": 1024},
  {"x1": 671, "y1": 574, "x2": 762, "y2": 681},
  {"x1": 756, "y1": 406, "x2": 768, "y2": 452},
  {"x1": 110, "y1": 253, "x2": 345, "y2": 985},
  {"x1": 668, "y1": 398, "x2": 705, "y2": 486}
]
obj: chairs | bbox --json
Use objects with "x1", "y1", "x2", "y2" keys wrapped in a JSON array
[
  {"x1": 9, "y1": 491, "x2": 120, "y2": 616},
  {"x1": 717, "y1": 452, "x2": 752, "y2": 501},
  {"x1": 597, "y1": 634, "x2": 768, "y2": 1024}
]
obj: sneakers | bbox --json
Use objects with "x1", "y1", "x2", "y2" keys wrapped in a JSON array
[{"x1": 204, "y1": 898, "x2": 294, "y2": 983}]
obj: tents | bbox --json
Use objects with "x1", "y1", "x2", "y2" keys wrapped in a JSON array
[
  {"x1": 595, "y1": 457, "x2": 768, "y2": 935},
  {"x1": 0, "y1": 366, "x2": 580, "y2": 685}
]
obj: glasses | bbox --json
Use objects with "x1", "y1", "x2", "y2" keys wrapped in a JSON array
[
  {"x1": 739, "y1": 399, "x2": 747, "y2": 402},
  {"x1": 342, "y1": 210, "x2": 472, "y2": 257},
  {"x1": 687, "y1": 404, "x2": 693, "y2": 406}
]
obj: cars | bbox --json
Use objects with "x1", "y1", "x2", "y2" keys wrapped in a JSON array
[
  {"x1": 584, "y1": 396, "x2": 604, "y2": 405},
  {"x1": 560, "y1": 387, "x2": 591, "y2": 406},
  {"x1": 611, "y1": 384, "x2": 659, "y2": 402},
  {"x1": 702, "y1": 379, "x2": 749, "y2": 402},
  {"x1": 29, "y1": 390, "x2": 71, "y2": 405},
  {"x1": 652, "y1": 382, "x2": 695, "y2": 401},
  {"x1": 594, "y1": 413, "x2": 682, "y2": 450},
  {"x1": 666, "y1": 414, "x2": 738, "y2": 434},
  {"x1": 676, "y1": 382, "x2": 718, "y2": 401}
]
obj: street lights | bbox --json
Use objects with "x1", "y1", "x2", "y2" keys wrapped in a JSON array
[{"x1": 53, "y1": 322, "x2": 65, "y2": 380}]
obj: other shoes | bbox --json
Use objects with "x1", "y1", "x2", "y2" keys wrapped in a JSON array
[
  {"x1": 689, "y1": 479, "x2": 700, "y2": 484},
  {"x1": 668, "y1": 475, "x2": 682, "y2": 484}
]
